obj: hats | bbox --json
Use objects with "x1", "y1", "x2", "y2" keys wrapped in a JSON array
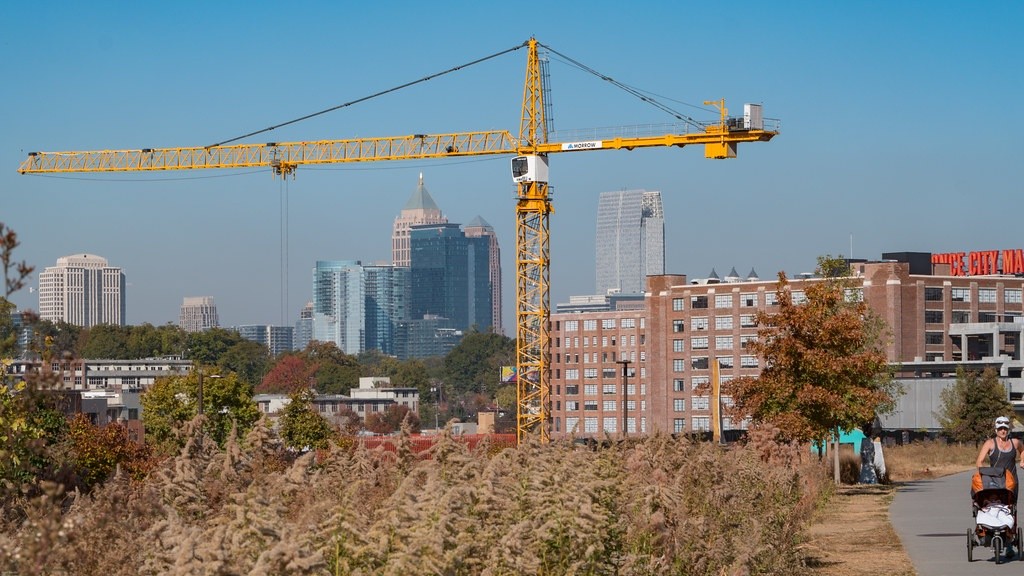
[{"x1": 995, "y1": 416, "x2": 1009, "y2": 429}]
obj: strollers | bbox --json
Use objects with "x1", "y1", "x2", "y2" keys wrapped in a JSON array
[{"x1": 965, "y1": 468, "x2": 1024, "y2": 565}]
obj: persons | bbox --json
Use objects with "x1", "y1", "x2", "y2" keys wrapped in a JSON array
[{"x1": 977, "y1": 417, "x2": 1024, "y2": 558}]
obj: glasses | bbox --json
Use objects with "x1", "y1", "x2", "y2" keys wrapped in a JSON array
[{"x1": 995, "y1": 421, "x2": 1010, "y2": 425}]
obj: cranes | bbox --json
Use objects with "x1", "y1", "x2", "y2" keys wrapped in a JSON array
[{"x1": 19, "y1": 35, "x2": 782, "y2": 451}]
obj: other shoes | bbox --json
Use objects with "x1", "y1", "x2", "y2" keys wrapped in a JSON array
[{"x1": 1006, "y1": 549, "x2": 1015, "y2": 558}]
